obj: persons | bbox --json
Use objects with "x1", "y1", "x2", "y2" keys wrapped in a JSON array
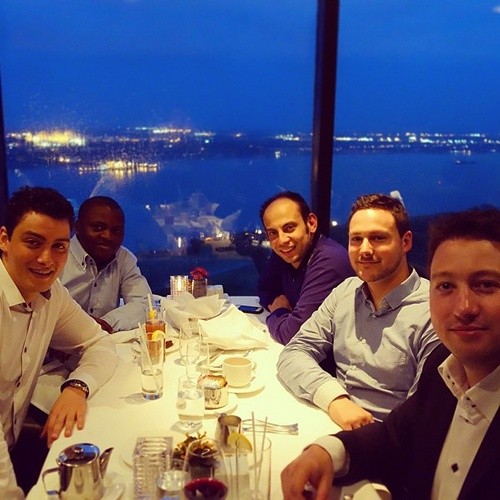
[
  {"x1": 28, "y1": 195, "x2": 155, "y2": 427},
  {"x1": 276, "y1": 194, "x2": 443, "y2": 431},
  {"x1": 1, "y1": 185, "x2": 120, "y2": 500},
  {"x1": 257, "y1": 190, "x2": 357, "y2": 377},
  {"x1": 280, "y1": 204, "x2": 499, "y2": 500}
]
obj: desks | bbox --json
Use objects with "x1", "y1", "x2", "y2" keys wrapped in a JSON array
[{"x1": 25, "y1": 297, "x2": 391, "y2": 500}]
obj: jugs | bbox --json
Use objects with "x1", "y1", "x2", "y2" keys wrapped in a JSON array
[{"x1": 43, "y1": 443, "x2": 114, "y2": 500}]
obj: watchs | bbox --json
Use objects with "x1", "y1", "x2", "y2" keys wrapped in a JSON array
[{"x1": 61, "y1": 381, "x2": 89, "y2": 399}]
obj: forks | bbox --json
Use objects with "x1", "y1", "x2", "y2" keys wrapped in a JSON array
[{"x1": 242, "y1": 419, "x2": 299, "y2": 432}]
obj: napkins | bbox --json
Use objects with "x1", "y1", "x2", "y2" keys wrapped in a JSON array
[
  {"x1": 30, "y1": 359, "x2": 70, "y2": 413},
  {"x1": 160, "y1": 294, "x2": 270, "y2": 350}
]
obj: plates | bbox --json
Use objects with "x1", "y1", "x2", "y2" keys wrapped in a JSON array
[
  {"x1": 49, "y1": 473, "x2": 124, "y2": 500},
  {"x1": 205, "y1": 393, "x2": 238, "y2": 414},
  {"x1": 133, "y1": 338, "x2": 182, "y2": 352},
  {"x1": 228, "y1": 370, "x2": 265, "y2": 393}
]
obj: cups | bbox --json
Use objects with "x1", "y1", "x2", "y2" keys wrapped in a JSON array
[
  {"x1": 158, "y1": 459, "x2": 190, "y2": 495},
  {"x1": 145, "y1": 306, "x2": 165, "y2": 340},
  {"x1": 185, "y1": 342, "x2": 209, "y2": 387},
  {"x1": 176, "y1": 376, "x2": 205, "y2": 431},
  {"x1": 180, "y1": 323, "x2": 201, "y2": 364},
  {"x1": 183, "y1": 439, "x2": 232, "y2": 500},
  {"x1": 192, "y1": 278, "x2": 207, "y2": 299},
  {"x1": 184, "y1": 479, "x2": 228, "y2": 500},
  {"x1": 223, "y1": 357, "x2": 256, "y2": 387},
  {"x1": 197, "y1": 375, "x2": 228, "y2": 409},
  {"x1": 140, "y1": 332, "x2": 165, "y2": 399},
  {"x1": 169, "y1": 275, "x2": 188, "y2": 300},
  {"x1": 134, "y1": 436, "x2": 173, "y2": 500},
  {"x1": 215, "y1": 414, "x2": 241, "y2": 453},
  {"x1": 236, "y1": 434, "x2": 272, "y2": 500}
]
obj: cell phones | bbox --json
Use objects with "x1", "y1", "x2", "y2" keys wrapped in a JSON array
[{"x1": 238, "y1": 305, "x2": 264, "y2": 313}]
